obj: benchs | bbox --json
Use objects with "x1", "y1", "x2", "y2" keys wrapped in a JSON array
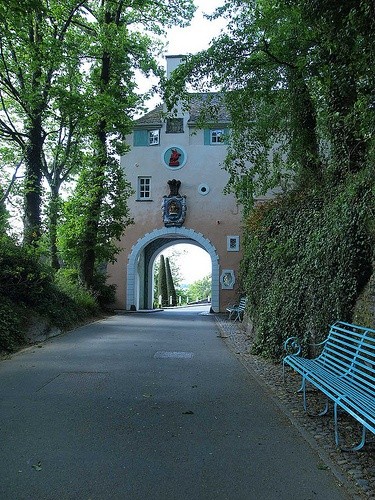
[
  {"x1": 225, "y1": 298, "x2": 245, "y2": 323},
  {"x1": 282, "y1": 321, "x2": 375, "y2": 451}
]
